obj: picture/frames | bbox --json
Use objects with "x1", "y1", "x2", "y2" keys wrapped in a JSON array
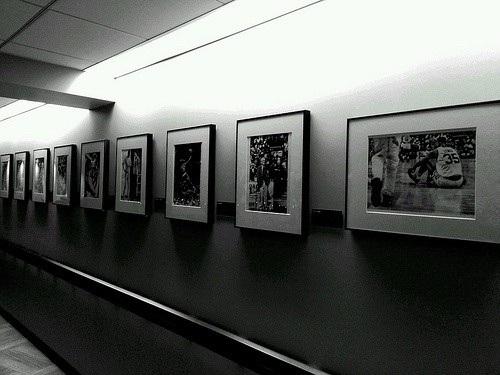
[
  {"x1": 113, "y1": 133, "x2": 153, "y2": 217},
  {"x1": 164, "y1": 123, "x2": 216, "y2": 224},
  {"x1": 31, "y1": 148, "x2": 50, "y2": 204},
  {"x1": 78, "y1": 139, "x2": 110, "y2": 211},
  {"x1": 13, "y1": 151, "x2": 30, "y2": 201},
  {"x1": 233, "y1": 110, "x2": 310, "y2": 240},
  {"x1": 52, "y1": 144, "x2": 77, "y2": 207},
  {"x1": 0, "y1": 153, "x2": 13, "y2": 200},
  {"x1": 343, "y1": 100, "x2": 500, "y2": 253}
]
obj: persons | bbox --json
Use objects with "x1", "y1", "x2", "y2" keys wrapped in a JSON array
[
  {"x1": 178, "y1": 155, "x2": 199, "y2": 206},
  {"x1": 84, "y1": 153, "x2": 98, "y2": 198},
  {"x1": 399, "y1": 130, "x2": 476, "y2": 162},
  {"x1": 407, "y1": 137, "x2": 464, "y2": 184},
  {"x1": 122, "y1": 150, "x2": 140, "y2": 199},
  {"x1": 371, "y1": 135, "x2": 403, "y2": 207},
  {"x1": 250, "y1": 135, "x2": 287, "y2": 208}
]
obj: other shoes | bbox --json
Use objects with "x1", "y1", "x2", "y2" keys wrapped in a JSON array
[
  {"x1": 370, "y1": 179, "x2": 381, "y2": 206},
  {"x1": 409, "y1": 172, "x2": 420, "y2": 184},
  {"x1": 383, "y1": 195, "x2": 393, "y2": 205}
]
